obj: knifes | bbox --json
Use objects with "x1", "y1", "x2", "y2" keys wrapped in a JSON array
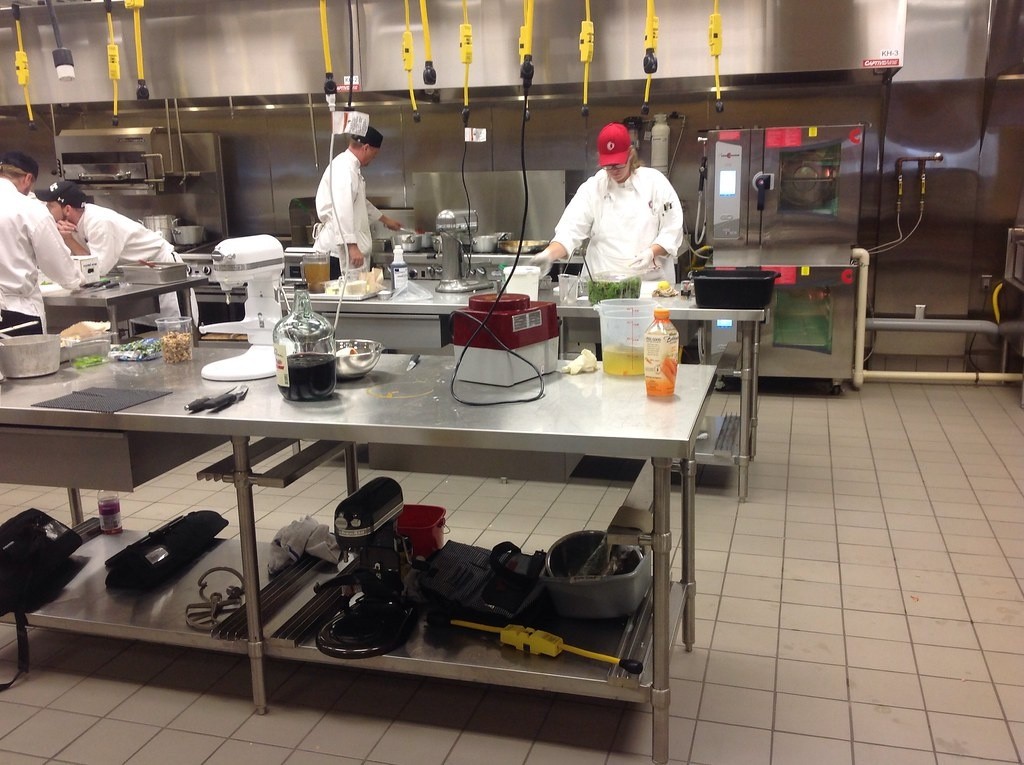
[
  {"x1": 406, "y1": 352, "x2": 420, "y2": 372},
  {"x1": 184, "y1": 384, "x2": 247, "y2": 411}
]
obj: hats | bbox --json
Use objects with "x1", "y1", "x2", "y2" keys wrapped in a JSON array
[
  {"x1": 351, "y1": 126, "x2": 383, "y2": 148},
  {"x1": 596, "y1": 123, "x2": 631, "y2": 167},
  {"x1": 0, "y1": 151, "x2": 38, "y2": 176},
  {"x1": 34, "y1": 181, "x2": 82, "y2": 207}
]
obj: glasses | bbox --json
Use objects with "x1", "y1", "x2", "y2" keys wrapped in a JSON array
[{"x1": 601, "y1": 149, "x2": 631, "y2": 170}]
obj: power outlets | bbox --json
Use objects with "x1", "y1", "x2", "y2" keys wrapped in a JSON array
[{"x1": 981, "y1": 275, "x2": 993, "y2": 291}]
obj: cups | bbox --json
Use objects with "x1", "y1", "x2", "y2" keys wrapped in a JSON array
[
  {"x1": 337, "y1": 266, "x2": 367, "y2": 296},
  {"x1": 155, "y1": 316, "x2": 193, "y2": 365},
  {"x1": 558, "y1": 274, "x2": 578, "y2": 303}
]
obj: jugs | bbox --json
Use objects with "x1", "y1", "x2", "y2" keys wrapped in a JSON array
[
  {"x1": 593, "y1": 297, "x2": 658, "y2": 377},
  {"x1": 299, "y1": 249, "x2": 331, "y2": 294}
]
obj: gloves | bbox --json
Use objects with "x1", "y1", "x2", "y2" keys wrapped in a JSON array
[
  {"x1": 528, "y1": 248, "x2": 554, "y2": 280},
  {"x1": 629, "y1": 247, "x2": 654, "y2": 271}
]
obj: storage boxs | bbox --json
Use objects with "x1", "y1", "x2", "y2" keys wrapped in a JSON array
[
  {"x1": 538, "y1": 544, "x2": 653, "y2": 620},
  {"x1": 393, "y1": 503, "x2": 451, "y2": 561},
  {"x1": 687, "y1": 267, "x2": 781, "y2": 310}
]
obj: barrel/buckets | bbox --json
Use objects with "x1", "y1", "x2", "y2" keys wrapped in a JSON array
[
  {"x1": 396, "y1": 504, "x2": 450, "y2": 560},
  {"x1": 503, "y1": 265, "x2": 540, "y2": 302}
]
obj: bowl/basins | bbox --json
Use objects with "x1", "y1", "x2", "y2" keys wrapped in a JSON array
[
  {"x1": 0, "y1": 333, "x2": 61, "y2": 378},
  {"x1": 499, "y1": 240, "x2": 549, "y2": 254},
  {"x1": 545, "y1": 530, "x2": 643, "y2": 578},
  {"x1": 335, "y1": 340, "x2": 384, "y2": 380}
]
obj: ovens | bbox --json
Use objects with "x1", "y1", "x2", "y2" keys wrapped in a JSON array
[{"x1": 705, "y1": 124, "x2": 864, "y2": 380}]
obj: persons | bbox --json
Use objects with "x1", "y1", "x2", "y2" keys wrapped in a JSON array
[
  {"x1": 311, "y1": 125, "x2": 406, "y2": 280},
  {"x1": 0, "y1": 151, "x2": 200, "y2": 339},
  {"x1": 529, "y1": 124, "x2": 683, "y2": 285}
]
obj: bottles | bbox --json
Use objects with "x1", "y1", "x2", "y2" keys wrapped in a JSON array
[
  {"x1": 390, "y1": 244, "x2": 408, "y2": 295},
  {"x1": 97, "y1": 489, "x2": 122, "y2": 535},
  {"x1": 644, "y1": 307, "x2": 679, "y2": 396},
  {"x1": 272, "y1": 282, "x2": 337, "y2": 401}
]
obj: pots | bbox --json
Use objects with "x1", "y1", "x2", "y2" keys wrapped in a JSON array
[
  {"x1": 390, "y1": 234, "x2": 422, "y2": 252},
  {"x1": 138, "y1": 215, "x2": 180, "y2": 244},
  {"x1": 170, "y1": 224, "x2": 204, "y2": 245},
  {"x1": 414, "y1": 231, "x2": 435, "y2": 248},
  {"x1": 431, "y1": 235, "x2": 442, "y2": 251},
  {"x1": 472, "y1": 235, "x2": 498, "y2": 253}
]
